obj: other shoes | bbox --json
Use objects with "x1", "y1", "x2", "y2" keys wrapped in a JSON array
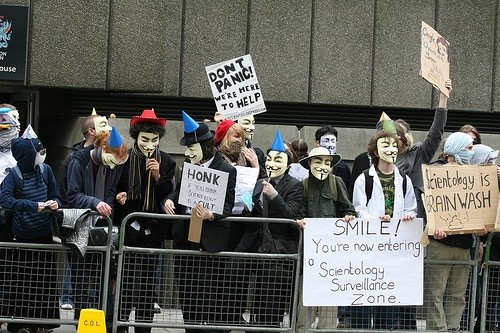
[
  {"x1": 18, "y1": 327, "x2": 32, "y2": 333},
  {"x1": 37, "y1": 328, "x2": 51, "y2": 333},
  {"x1": 337, "y1": 320, "x2": 351, "y2": 328},
  {"x1": 60, "y1": 300, "x2": 74, "y2": 309}
]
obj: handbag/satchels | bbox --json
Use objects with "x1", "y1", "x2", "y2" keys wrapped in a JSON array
[
  {"x1": 83, "y1": 213, "x2": 120, "y2": 247},
  {"x1": 260, "y1": 233, "x2": 300, "y2": 278}
]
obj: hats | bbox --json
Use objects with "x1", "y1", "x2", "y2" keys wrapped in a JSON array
[
  {"x1": 299, "y1": 147, "x2": 342, "y2": 169},
  {"x1": 212, "y1": 119, "x2": 236, "y2": 145},
  {"x1": 181, "y1": 110, "x2": 200, "y2": 133},
  {"x1": 271, "y1": 130, "x2": 285, "y2": 152},
  {"x1": 107, "y1": 126, "x2": 122, "y2": 148},
  {"x1": 179, "y1": 123, "x2": 212, "y2": 143},
  {"x1": 92, "y1": 107, "x2": 96, "y2": 115},
  {"x1": 130, "y1": 109, "x2": 166, "y2": 127}
]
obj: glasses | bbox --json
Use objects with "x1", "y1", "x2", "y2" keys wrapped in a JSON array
[{"x1": 40, "y1": 148, "x2": 47, "y2": 155}]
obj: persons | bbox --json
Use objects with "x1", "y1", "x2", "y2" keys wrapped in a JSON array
[{"x1": 0, "y1": 79, "x2": 500, "y2": 333}]
